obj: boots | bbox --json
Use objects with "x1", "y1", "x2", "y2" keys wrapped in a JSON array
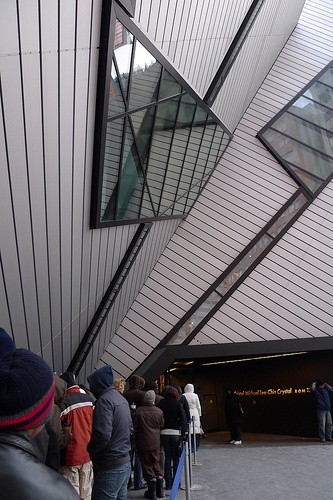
[
  {"x1": 144, "y1": 480, "x2": 157, "y2": 500},
  {"x1": 156, "y1": 478, "x2": 165, "y2": 497}
]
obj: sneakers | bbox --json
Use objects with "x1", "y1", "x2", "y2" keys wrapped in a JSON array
[
  {"x1": 234, "y1": 440, "x2": 242, "y2": 445},
  {"x1": 230, "y1": 440, "x2": 235, "y2": 443}
]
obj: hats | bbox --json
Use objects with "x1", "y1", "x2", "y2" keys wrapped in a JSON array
[
  {"x1": 0, "y1": 328, "x2": 15, "y2": 357},
  {"x1": 143, "y1": 390, "x2": 156, "y2": 404},
  {"x1": 59, "y1": 372, "x2": 76, "y2": 389},
  {"x1": 0, "y1": 349, "x2": 55, "y2": 432}
]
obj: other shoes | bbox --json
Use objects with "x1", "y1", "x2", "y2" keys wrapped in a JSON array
[
  {"x1": 326, "y1": 438, "x2": 332, "y2": 441},
  {"x1": 141, "y1": 485, "x2": 148, "y2": 489},
  {"x1": 320, "y1": 439, "x2": 325, "y2": 443}
]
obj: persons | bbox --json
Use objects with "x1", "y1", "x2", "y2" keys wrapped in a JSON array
[
  {"x1": 132, "y1": 390, "x2": 165, "y2": 500},
  {"x1": 0, "y1": 348, "x2": 84, "y2": 500},
  {"x1": 86, "y1": 365, "x2": 134, "y2": 500},
  {"x1": 311, "y1": 381, "x2": 333, "y2": 443},
  {"x1": 123, "y1": 376, "x2": 148, "y2": 490},
  {"x1": 223, "y1": 385, "x2": 245, "y2": 445},
  {"x1": 34, "y1": 372, "x2": 72, "y2": 473},
  {"x1": 158, "y1": 386, "x2": 187, "y2": 490},
  {"x1": 56, "y1": 371, "x2": 96, "y2": 500},
  {"x1": 182, "y1": 384, "x2": 202, "y2": 454}
]
